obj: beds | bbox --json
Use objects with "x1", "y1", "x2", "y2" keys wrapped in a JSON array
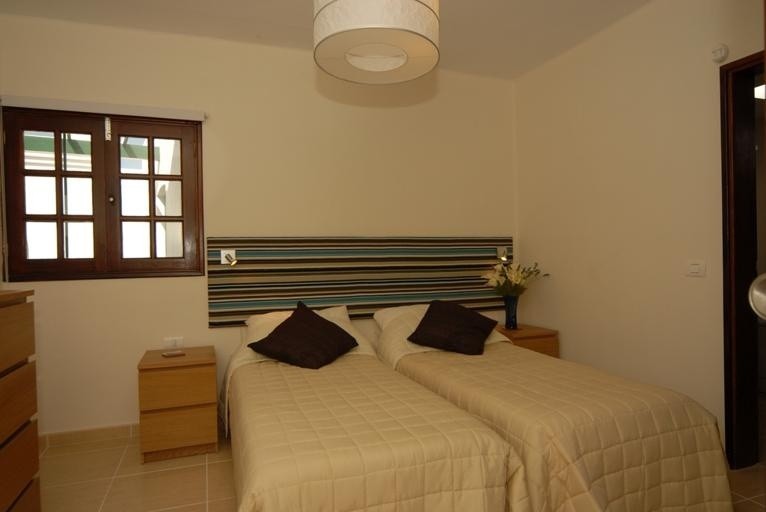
[
  {"x1": 221, "y1": 341, "x2": 528, "y2": 512},
  {"x1": 373, "y1": 331, "x2": 738, "y2": 512}
]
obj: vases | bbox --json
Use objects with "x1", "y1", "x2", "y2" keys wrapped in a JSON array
[{"x1": 502, "y1": 293, "x2": 519, "y2": 330}]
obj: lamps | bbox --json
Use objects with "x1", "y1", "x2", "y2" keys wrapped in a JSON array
[
  {"x1": 307, "y1": 0, "x2": 445, "y2": 86},
  {"x1": 220, "y1": 248, "x2": 237, "y2": 267},
  {"x1": 496, "y1": 245, "x2": 509, "y2": 261}
]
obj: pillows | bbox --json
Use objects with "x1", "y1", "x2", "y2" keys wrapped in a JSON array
[
  {"x1": 403, "y1": 296, "x2": 501, "y2": 357},
  {"x1": 370, "y1": 302, "x2": 430, "y2": 331},
  {"x1": 246, "y1": 297, "x2": 360, "y2": 373},
  {"x1": 243, "y1": 304, "x2": 354, "y2": 342}
]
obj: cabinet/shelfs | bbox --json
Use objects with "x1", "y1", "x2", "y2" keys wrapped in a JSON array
[{"x1": 1, "y1": 286, "x2": 47, "y2": 512}]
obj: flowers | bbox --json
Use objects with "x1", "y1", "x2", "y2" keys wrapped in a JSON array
[{"x1": 479, "y1": 255, "x2": 552, "y2": 291}]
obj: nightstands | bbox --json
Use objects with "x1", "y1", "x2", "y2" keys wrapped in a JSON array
[
  {"x1": 496, "y1": 320, "x2": 561, "y2": 360},
  {"x1": 135, "y1": 344, "x2": 222, "y2": 466}
]
